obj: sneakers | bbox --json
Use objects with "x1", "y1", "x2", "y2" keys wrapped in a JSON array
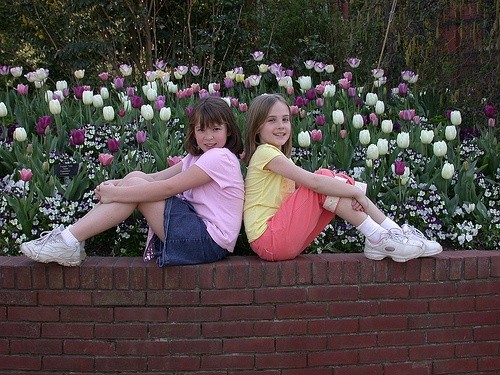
[
  {"x1": 385, "y1": 223, "x2": 443, "y2": 258},
  {"x1": 20, "y1": 224, "x2": 86, "y2": 266},
  {"x1": 363, "y1": 231, "x2": 426, "y2": 263}
]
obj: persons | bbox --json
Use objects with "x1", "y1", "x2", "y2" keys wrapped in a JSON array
[
  {"x1": 243, "y1": 93, "x2": 444, "y2": 262},
  {"x1": 20, "y1": 95, "x2": 245, "y2": 268}
]
